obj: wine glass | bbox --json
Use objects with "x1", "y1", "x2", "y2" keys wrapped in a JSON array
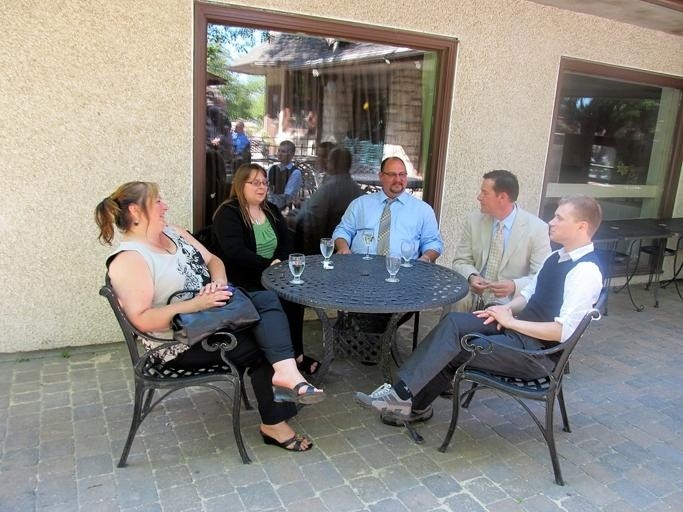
[
  {"x1": 383, "y1": 251, "x2": 401, "y2": 282},
  {"x1": 361, "y1": 229, "x2": 375, "y2": 261},
  {"x1": 400, "y1": 239, "x2": 415, "y2": 268},
  {"x1": 286, "y1": 253, "x2": 306, "y2": 285},
  {"x1": 318, "y1": 238, "x2": 334, "y2": 265}
]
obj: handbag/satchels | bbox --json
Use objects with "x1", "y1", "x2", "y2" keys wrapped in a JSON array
[{"x1": 167, "y1": 286, "x2": 262, "y2": 347}]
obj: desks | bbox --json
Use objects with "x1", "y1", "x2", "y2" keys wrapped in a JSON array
[
  {"x1": 593, "y1": 215, "x2": 683, "y2": 312},
  {"x1": 352, "y1": 175, "x2": 425, "y2": 196}
]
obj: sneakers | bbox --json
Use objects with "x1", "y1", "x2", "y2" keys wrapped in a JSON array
[
  {"x1": 352, "y1": 382, "x2": 412, "y2": 421},
  {"x1": 379, "y1": 404, "x2": 433, "y2": 427}
]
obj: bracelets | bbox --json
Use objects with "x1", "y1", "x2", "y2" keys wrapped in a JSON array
[
  {"x1": 421, "y1": 254, "x2": 433, "y2": 264},
  {"x1": 421, "y1": 255, "x2": 431, "y2": 264}
]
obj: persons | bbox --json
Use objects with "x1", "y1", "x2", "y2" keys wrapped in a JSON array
[
  {"x1": 209, "y1": 163, "x2": 321, "y2": 375},
  {"x1": 230, "y1": 122, "x2": 249, "y2": 156},
  {"x1": 216, "y1": 94, "x2": 232, "y2": 152},
  {"x1": 205, "y1": 87, "x2": 231, "y2": 156},
  {"x1": 441, "y1": 169, "x2": 553, "y2": 396},
  {"x1": 94, "y1": 180, "x2": 327, "y2": 451},
  {"x1": 352, "y1": 192, "x2": 604, "y2": 427},
  {"x1": 315, "y1": 142, "x2": 335, "y2": 179},
  {"x1": 305, "y1": 110, "x2": 317, "y2": 136},
  {"x1": 331, "y1": 157, "x2": 442, "y2": 263},
  {"x1": 266, "y1": 141, "x2": 301, "y2": 211},
  {"x1": 287, "y1": 146, "x2": 364, "y2": 247}
]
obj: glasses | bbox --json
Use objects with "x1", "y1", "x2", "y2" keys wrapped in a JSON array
[
  {"x1": 383, "y1": 171, "x2": 407, "y2": 177},
  {"x1": 246, "y1": 181, "x2": 269, "y2": 187}
]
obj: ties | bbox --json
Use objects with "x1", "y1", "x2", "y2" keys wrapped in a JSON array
[
  {"x1": 481, "y1": 222, "x2": 505, "y2": 308},
  {"x1": 377, "y1": 198, "x2": 396, "y2": 256}
]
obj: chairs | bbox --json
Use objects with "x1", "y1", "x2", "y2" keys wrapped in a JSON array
[
  {"x1": 285, "y1": 158, "x2": 323, "y2": 219},
  {"x1": 541, "y1": 202, "x2": 632, "y2": 318},
  {"x1": 96, "y1": 280, "x2": 258, "y2": 469},
  {"x1": 436, "y1": 283, "x2": 610, "y2": 487},
  {"x1": 343, "y1": 249, "x2": 425, "y2": 361}
]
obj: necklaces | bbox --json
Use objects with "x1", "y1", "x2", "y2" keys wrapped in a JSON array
[
  {"x1": 248, "y1": 206, "x2": 263, "y2": 226},
  {"x1": 141, "y1": 235, "x2": 172, "y2": 255}
]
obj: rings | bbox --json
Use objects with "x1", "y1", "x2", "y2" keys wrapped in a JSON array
[{"x1": 493, "y1": 312, "x2": 496, "y2": 317}]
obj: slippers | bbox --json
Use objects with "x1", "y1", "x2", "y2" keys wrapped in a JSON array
[
  {"x1": 297, "y1": 354, "x2": 321, "y2": 375},
  {"x1": 259, "y1": 430, "x2": 313, "y2": 452},
  {"x1": 271, "y1": 382, "x2": 326, "y2": 405}
]
